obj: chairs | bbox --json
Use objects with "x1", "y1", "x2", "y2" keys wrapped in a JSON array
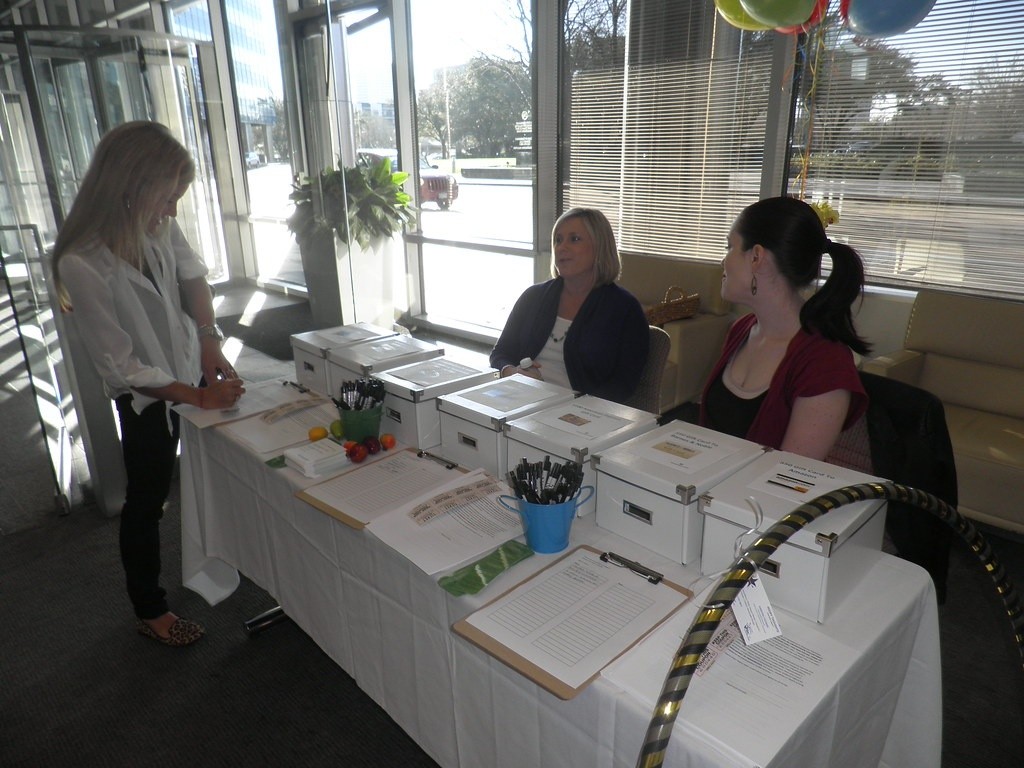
[
  {"x1": 631, "y1": 324, "x2": 673, "y2": 416},
  {"x1": 851, "y1": 371, "x2": 942, "y2": 492}
]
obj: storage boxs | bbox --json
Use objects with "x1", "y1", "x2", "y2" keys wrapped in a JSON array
[
  {"x1": 367, "y1": 352, "x2": 500, "y2": 453},
  {"x1": 326, "y1": 333, "x2": 445, "y2": 403},
  {"x1": 435, "y1": 372, "x2": 581, "y2": 482},
  {"x1": 289, "y1": 322, "x2": 400, "y2": 396},
  {"x1": 587, "y1": 417, "x2": 772, "y2": 567},
  {"x1": 701, "y1": 451, "x2": 894, "y2": 626},
  {"x1": 503, "y1": 392, "x2": 663, "y2": 519}
]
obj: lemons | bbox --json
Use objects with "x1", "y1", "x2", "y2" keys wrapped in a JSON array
[{"x1": 309, "y1": 426, "x2": 328, "y2": 441}]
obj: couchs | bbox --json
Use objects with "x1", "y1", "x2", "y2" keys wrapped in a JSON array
[
  {"x1": 616, "y1": 253, "x2": 741, "y2": 413},
  {"x1": 860, "y1": 288, "x2": 1024, "y2": 525}
]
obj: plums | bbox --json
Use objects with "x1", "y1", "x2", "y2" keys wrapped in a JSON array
[{"x1": 381, "y1": 434, "x2": 396, "y2": 449}]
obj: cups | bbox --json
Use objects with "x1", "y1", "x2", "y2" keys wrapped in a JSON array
[
  {"x1": 498, "y1": 485, "x2": 595, "y2": 554},
  {"x1": 337, "y1": 400, "x2": 384, "y2": 445}
]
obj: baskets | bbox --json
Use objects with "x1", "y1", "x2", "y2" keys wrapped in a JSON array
[{"x1": 645, "y1": 286, "x2": 700, "y2": 326}]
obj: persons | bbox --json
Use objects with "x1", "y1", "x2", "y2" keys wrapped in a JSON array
[
  {"x1": 489, "y1": 208, "x2": 651, "y2": 405},
  {"x1": 50, "y1": 121, "x2": 247, "y2": 646},
  {"x1": 699, "y1": 197, "x2": 876, "y2": 477}
]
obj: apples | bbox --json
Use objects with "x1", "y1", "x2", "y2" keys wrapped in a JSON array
[{"x1": 343, "y1": 439, "x2": 381, "y2": 461}]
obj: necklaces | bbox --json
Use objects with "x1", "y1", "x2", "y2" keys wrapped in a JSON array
[{"x1": 550, "y1": 332, "x2": 568, "y2": 343}]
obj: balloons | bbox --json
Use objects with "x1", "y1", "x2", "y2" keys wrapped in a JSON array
[{"x1": 713, "y1": 0, "x2": 936, "y2": 38}]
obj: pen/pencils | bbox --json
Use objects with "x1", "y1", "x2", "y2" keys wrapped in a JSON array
[
  {"x1": 511, "y1": 453, "x2": 584, "y2": 505},
  {"x1": 332, "y1": 377, "x2": 386, "y2": 410},
  {"x1": 215, "y1": 366, "x2": 227, "y2": 380}
]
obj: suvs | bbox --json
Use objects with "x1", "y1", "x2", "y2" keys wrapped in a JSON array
[{"x1": 356, "y1": 147, "x2": 459, "y2": 209}]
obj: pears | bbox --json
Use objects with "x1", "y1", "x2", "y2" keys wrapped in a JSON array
[{"x1": 330, "y1": 420, "x2": 344, "y2": 440}]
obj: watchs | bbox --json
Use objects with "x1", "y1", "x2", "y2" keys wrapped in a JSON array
[{"x1": 198, "y1": 324, "x2": 224, "y2": 341}]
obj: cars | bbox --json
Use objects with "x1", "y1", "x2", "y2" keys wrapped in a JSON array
[{"x1": 244, "y1": 152, "x2": 261, "y2": 170}]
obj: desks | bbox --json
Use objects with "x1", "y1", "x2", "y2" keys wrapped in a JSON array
[{"x1": 181, "y1": 371, "x2": 943, "y2": 767}]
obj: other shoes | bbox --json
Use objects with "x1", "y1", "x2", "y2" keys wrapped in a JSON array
[{"x1": 136, "y1": 609, "x2": 205, "y2": 648}]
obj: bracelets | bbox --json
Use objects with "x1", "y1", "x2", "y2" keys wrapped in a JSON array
[{"x1": 199, "y1": 387, "x2": 204, "y2": 408}]
